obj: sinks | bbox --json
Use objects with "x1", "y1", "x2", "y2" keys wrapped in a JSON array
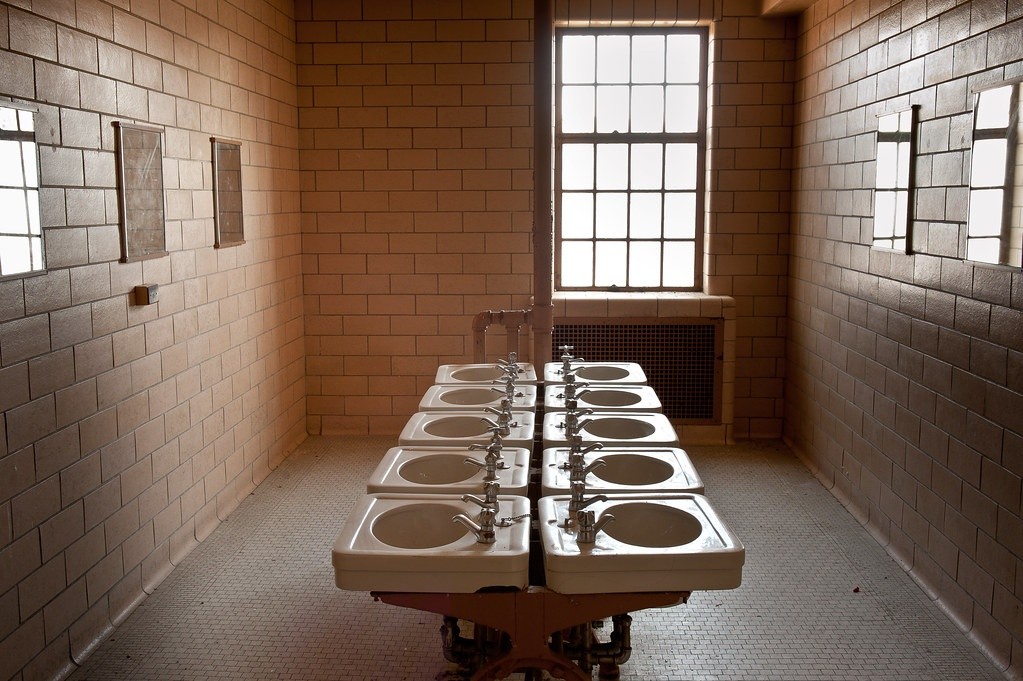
[
  {"x1": 367, "y1": 446, "x2": 530, "y2": 497},
  {"x1": 399, "y1": 411, "x2": 536, "y2": 467},
  {"x1": 538, "y1": 492, "x2": 745, "y2": 595},
  {"x1": 332, "y1": 494, "x2": 529, "y2": 593},
  {"x1": 544, "y1": 362, "x2": 647, "y2": 386},
  {"x1": 544, "y1": 385, "x2": 663, "y2": 412},
  {"x1": 541, "y1": 447, "x2": 704, "y2": 496},
  {"x1": 436, "y1": 363, "x2": 537, "y2": 386},
  {"x1": 419, "y1": 385, "x2": 537, "y2": 411},
  {"x1": 543, "y1": 411, "x2": 679, "y2": 449}
]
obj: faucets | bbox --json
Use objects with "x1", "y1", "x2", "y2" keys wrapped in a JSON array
[
  {"x1": 565, "y1": 384, "x2": 592, "y2": 404},
  {"x1": 468, "y1": 427, "x2": 505, "y2": 459},
  {"x1": 561, "y1": 356, "x2": 587, "y2": 379},
  {"x1": 577, "y1": 510, "x2": 615, "y2": 543},
  {"x1": 559, "y1": 344, "x2": 585, "y2": 362},
  {"x1": 569, "y1": 434, "x2": 603, "y2": 461},
  {"x1": 565, "y1": 399, "x2": 593, "y2": 417},
  {"x1": 566, "y1": 374, "x2": 589, "y2": 389},
  {"x1": 480, "y1": 412, "x2": 511, "y2": 435},
  {"x1": 570, "y1": 452, "x2": 608, "y2": 481},
  {"x1": 569, "y1": 480, "x2": 608, "y2": 511},
  {"x1": 495, "y1": 363, "x2": 520, "y2": 379},
  {"x1": 496, "y1": 352, "x2": 525, "y2": 373},
  {"x1": 490, "y1": 376, "x2": 519, "y2": 402},
  {"x1": 566, "y1": 412, "x2": 593, "y2": 439},
  {"x1": 463, "y1": 443, "x2": 501, "y2": 480},
  {"x1": 484, "y1": 399, "x2": 512, "y2": 420},
  {"x1": 452, "y1": 508, "x2": 496, "y2": 543},
  {"x1": 461, "y1": 481, "x2": 500, "y2": 512}
]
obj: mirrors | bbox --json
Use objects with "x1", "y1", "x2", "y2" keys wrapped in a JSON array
[
  {"x1": 111, "y1": 120, "x2": 169, "y2": 264},
  {"x1": 870, "y1": 103, "x2": 920, "y2": 255},
  {"x1": 210, "y1": 136, "x2": 246, "y2": 249},
  {"x1": 963, "y1": 78, "x2": 1023, "y2": 274},
  {"x1": 0, "y1": 100, "x2": 48, "y2": 282}
]
